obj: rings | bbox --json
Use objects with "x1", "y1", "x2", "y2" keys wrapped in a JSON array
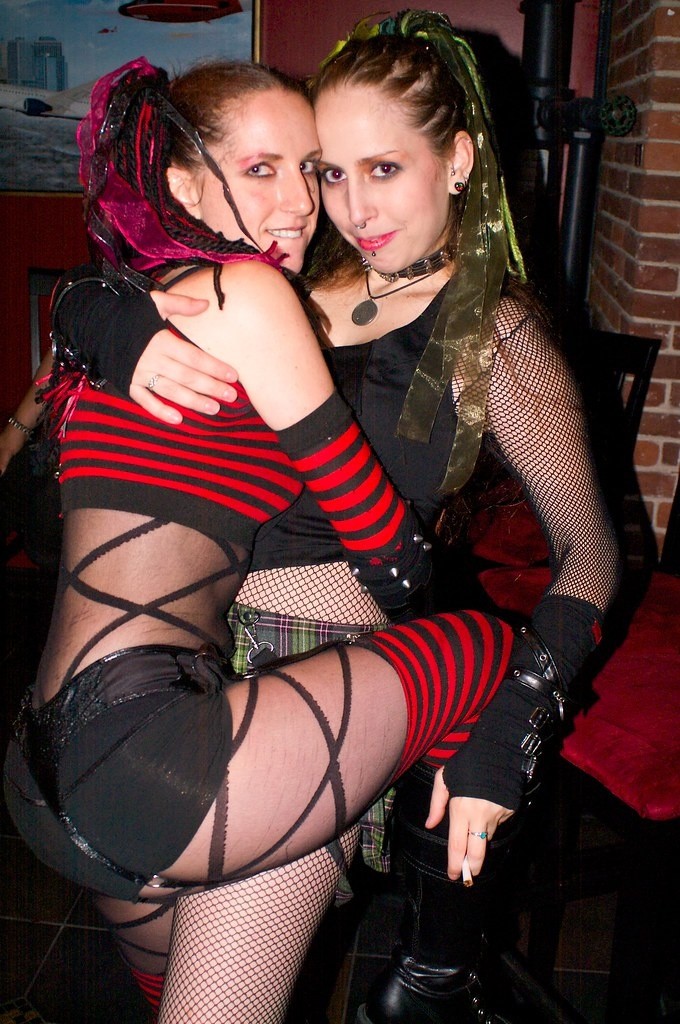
[
  {"x1": 148, "y1": 374, "x2": 160, "y2": 391},
  {"x1": 470, "y1": 832, "x2": 489, "y2": 838}
]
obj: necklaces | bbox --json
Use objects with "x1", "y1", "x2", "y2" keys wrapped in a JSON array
[
  {"x1": 350, "y1": 264, "x2": 430, "y2": 326},
  {"x1": 375, "y1": 240, "x2": 458, "y2": 283}
]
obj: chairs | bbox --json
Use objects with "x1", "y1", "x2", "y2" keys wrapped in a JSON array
[
  {"x1": 427, "y1": 329, "x2": 664, "y2": 611},
  {"x1": 499, "y1": 474, "x2": 679, "y2": 1023},
  {"x1": 25, "y1": 267, "x2": 67, "y2": 646}
]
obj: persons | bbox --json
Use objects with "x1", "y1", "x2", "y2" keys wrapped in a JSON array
[
  {"x1": 0, "y1": 346, "x2": 61, "y2": 472},
  {"x1": 0, "y1": 55, "x2": 544, "y2": 1023},
  {"x1": 48, "y1": 8, "x2": 620, "y2": 1023}
]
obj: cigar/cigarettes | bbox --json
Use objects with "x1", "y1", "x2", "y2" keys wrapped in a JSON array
[{"x1": 462, "y1": 858, "x2": 473, "y2": 887}]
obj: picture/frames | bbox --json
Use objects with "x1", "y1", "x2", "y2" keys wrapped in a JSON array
[{"x1": 0, "y1": 1, "x2": 260, "y2": 198}]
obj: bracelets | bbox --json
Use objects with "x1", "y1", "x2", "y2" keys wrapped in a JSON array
[{"x1": 7, "y1": 416, "x2": 34, "y2": 439}]
